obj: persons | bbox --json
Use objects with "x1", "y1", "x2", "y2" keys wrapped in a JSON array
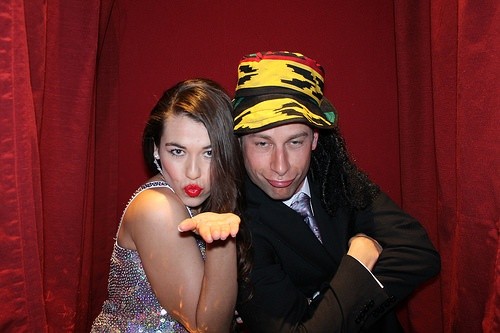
[
  {"x1": 88, "y1": 77, "x2": 243, "y2": 333},
  {"x1": 227, "y1": 50, "x2": 442, "y2": 332}
]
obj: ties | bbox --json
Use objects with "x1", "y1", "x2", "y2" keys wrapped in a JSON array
[{"x1": 290, "y1": 192, "x2": 323, "y2": 245}]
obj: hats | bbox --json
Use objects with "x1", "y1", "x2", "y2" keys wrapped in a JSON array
[{"x1": 232, "y1": 51, "x2": 338, "y2": 134}]
